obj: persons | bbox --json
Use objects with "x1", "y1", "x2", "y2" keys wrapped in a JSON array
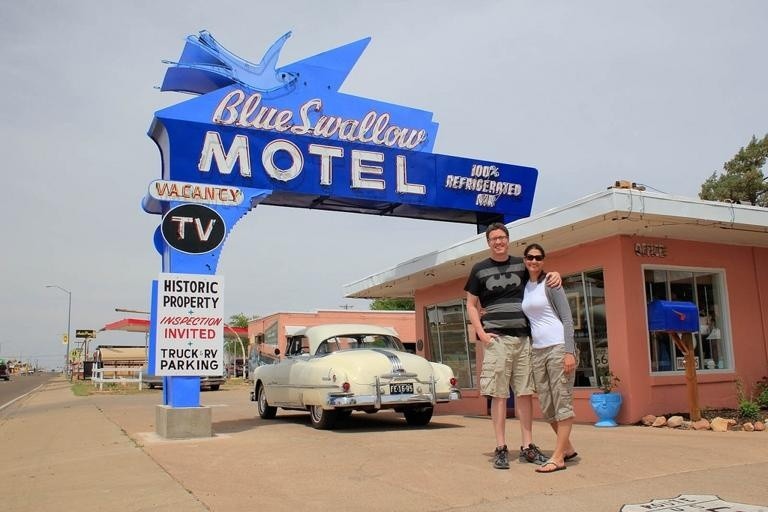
[
  {"x1": 477, "y1": 243, "x2": 581, "y2": 474},
  {"x1": 462, "y1": 222, "x2": 563, "y2": 470}
]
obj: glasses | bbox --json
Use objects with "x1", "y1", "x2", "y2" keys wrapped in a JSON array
[
  {"x1": 525, "y1": 254, "x2": 545, "y2": 261},
  {"x1": 487, "y1": 236, "x2": 508, "y2": 241}
]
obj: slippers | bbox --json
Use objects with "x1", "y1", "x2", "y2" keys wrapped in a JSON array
[{"x1": 535, "y1": 452, "x2": 578, "y2": 472}]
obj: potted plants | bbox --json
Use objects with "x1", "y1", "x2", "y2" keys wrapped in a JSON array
[{"x1": 589, "y1": 367, "x2": 623, "y2": 428}]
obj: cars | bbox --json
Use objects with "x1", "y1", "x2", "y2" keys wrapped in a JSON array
[
  {"x1": 431, "y1": 313, "x2": 475, "y2": 354},
  {"x1": 226, "y1": 358, "x2": 249, "y2": 379},
  {"x1": 140, "y1": 361, "x2": 226, "y2": 391},
  {"x1": 0, "y1": 364, "x2": 10, "y2": 380},
  {"x1": 249, "y1": 324, "x2": 461, "y2": 431}
]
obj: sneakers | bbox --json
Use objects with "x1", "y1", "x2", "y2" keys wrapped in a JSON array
[
  {"x1": 519, "y1": 443, "x2": 551, "y2": 465},
  {"x1": 493, "y1": 444, "x2": 510, "y2": 469}
]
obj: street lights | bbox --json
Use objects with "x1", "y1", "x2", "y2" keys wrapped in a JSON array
[{"x1": 45, "y1": 284, "x2": 72, "y2": 379}]
